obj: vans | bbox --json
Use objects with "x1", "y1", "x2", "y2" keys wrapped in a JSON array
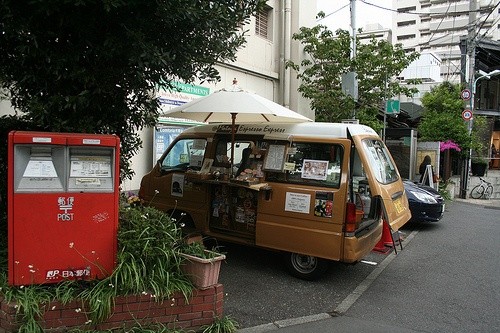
[{"x1": 135, "y1": 122, "x2": 413, "y2": 282}]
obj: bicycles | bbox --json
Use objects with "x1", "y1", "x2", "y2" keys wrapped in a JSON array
[{"x1": 471, "y1": 176, "x2": 493, "y2": 200}]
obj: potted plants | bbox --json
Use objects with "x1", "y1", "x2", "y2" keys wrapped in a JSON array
[{"x1": 180, "y1": 237, "x2": 227, "y2": 291}]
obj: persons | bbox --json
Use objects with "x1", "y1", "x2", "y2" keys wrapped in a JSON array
[{"x1": 419, "y1": 155, "x2": 438, "y2": 186}]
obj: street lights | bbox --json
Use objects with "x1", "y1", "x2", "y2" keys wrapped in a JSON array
[{"x1": 463, "y1": 69, "x2": 500, "y2": 199}]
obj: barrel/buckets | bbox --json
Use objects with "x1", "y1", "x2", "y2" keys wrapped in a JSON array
[
  {"x1": 355, "y1": 209, "x2": 364, "y2": 229},
  {"x1": 344, "y1": 203, "x2": 356, "y2": 232}
]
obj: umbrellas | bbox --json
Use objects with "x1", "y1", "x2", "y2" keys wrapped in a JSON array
[{"x1": 158, "y1": 79, "x2": 313, "y2": 177}]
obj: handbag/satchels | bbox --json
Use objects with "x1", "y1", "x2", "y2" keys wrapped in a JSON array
[{"x1": 433, "y1": 175, "x2": 439, "y2": 183}]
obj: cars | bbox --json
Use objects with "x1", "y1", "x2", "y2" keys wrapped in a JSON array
[{"x1": 401, "y1": 177, "x2": 446, "y2": 225}]
